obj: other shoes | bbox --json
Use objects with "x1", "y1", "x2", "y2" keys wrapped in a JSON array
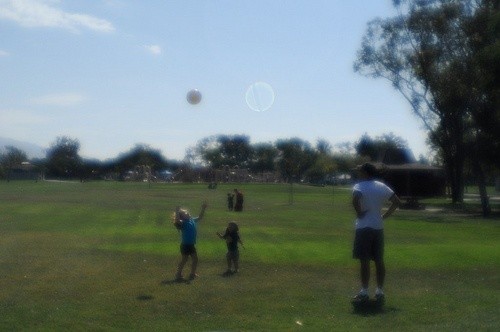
[
  {"x1": 371, "y1": 292, "x2": 384, "y2": 302},
  {"x1": 351, "y1": 294, "x2": 369, "y2": 302}
]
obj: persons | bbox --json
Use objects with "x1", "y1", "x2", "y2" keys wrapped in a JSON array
[
  {"x1": 352, "y1": 163, "x2": 401, "y2": 302},
  {"x1": 483, "y1": 195, "x2": 492, "y2": 218},
  {"x1": 152, "y1": 174, "x2": 219, "y2": 190},
  {"x1": 216, "y1": 222, "x2": 245, "y2": 276},
  {"x1": 226, "y1": 188, "x2": 244, "y2": 211},
  {"x1": 79, "y1": 173, "x2": 89, "y2": 185},
  {"x1": 173, "y1": 198, "x2": 208, "y2": 282}
]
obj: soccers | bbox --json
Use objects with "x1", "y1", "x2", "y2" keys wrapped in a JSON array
[{"x1": 186, "y1": 90, "x2": 202, "y2": 105}]
